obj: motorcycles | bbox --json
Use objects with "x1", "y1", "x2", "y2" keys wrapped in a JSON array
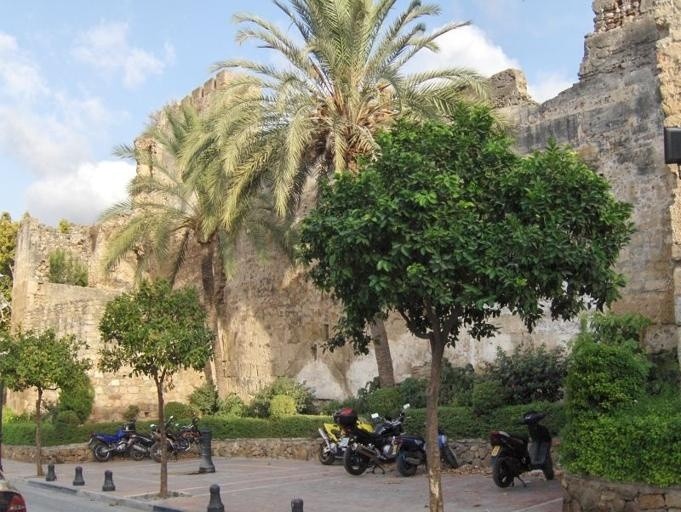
[
  {"x1": 332, "y1": 400, "x2": 413, "y2": 477},
  {"x1": 88, "y1": 420, "x2": 145, "y2": 461},
  {"x1": 130, "y1": 423, "x2": 173, "y2": 461},
  {"x1": 395, "y1": 421, "x2": 459, "y2": 479},
  {"x1": 150, "y1": 411, "x2": 207, "y2": 462},
  {"x1": 490, "y1": 408, "x2": 558, "y2": 489},
  {"x1": 318, "y1": 418, "x2": 396, "y2": 465}
]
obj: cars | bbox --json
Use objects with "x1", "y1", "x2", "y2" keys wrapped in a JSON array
[{"x1": 0, "y1": 459, "x2": 26, "y2": 512}]
